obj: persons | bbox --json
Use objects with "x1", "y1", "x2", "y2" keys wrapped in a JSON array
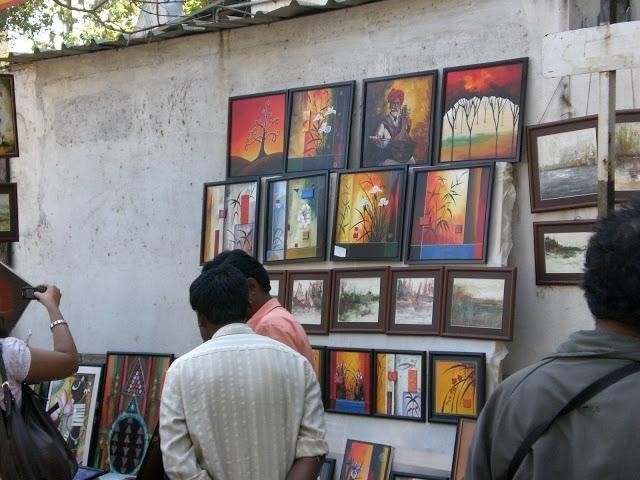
[
  {"x1": 202, "y1": 250, "x2": 313, "y2": 367},
  {"x1": 375, "y1": 88, "x2": 416, "y2": 166},
  {"x1": 160, "y1": 263, "x2": 329, "y2": 480},
  {"x1": 0, "y1": 283, "x2": 79, "y2": 412},
  {"x1": 466, "y1": 209, "x2": 640, "y2": 480}
]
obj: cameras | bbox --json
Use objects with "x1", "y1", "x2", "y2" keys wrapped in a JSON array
[{"x1": 21, "y1": 288, "x2": 46, "y2": 300}]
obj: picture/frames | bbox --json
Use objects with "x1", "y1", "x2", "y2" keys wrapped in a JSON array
[
  {"x1": 1, "y1": 262, "x2": 35, "y2": 341},
  {"x1": 265, "y1": 270, "x2": 286, "y2": 310},
  {"x1": 385, "y1": 265, "x2": 443, "y2": 336},
  {"x1": 428, "y1": 350, "x2": 486, "y2": 425},
  {"x1": 329, "y1": 266, "x2": 390, "y2": 334},
  {"x1": 262, "y1": 170, "x2": 329, "y2": 266},
  {"x1": 324, "y1": 346, "x2": 373, "y2": 417},
  {"x1": 226, "y1": 89, "x2": 289, "y2": 181},
  {"x1": 403, "y1": 161, "x2": 496, "y2": 265},
  {"x1": 526, "y1": 114, "x2": 599, "y2": 214},
  {"x1": 0, "y1": 183, "x2": 20, "y2": 243},
  {"x1": 0, "y1": 73, "x2": 19, "y2": 159},
  {"x1": 330, "y1": 166, "x2": 407, "y2": 262},
  {"x1": 437, "y1": 58, "x2": 529, "y2": 164},
  {"x1": 284, "y1": 80, "x2": 355, "y2": 172},
  {"x1": 440, "y1": 266, "x2": 517, "y2": 342},
  {"x1": 310, "y1": 345, "x2": 326, "y2": 404},
  {"x1": 85, "y1": 350, "x2": 175, "y2": 477},
  {"x1": 452, "y1": 416, "x2": 479, "y2": 480},
  {"x1": 373, "y1": 349, "x2": 427, "y2": 423},
  {"x1": 285, "y1": 269, "x2": 332, "y2": 336},
  {"x1": 337, "y1": 438, "x2": 394, "y2": 480},
  {"x1": 532, "y1": 219, "x2": 599, "y2": 286},
  {"x1": 609, "y1": 109, "x2": 640, "y2": 203},
  {"x1": 200, "y1": 176, "x2": 261, "y2": 267},
  {"x1": 359, "y1": 69, "x2": 438, "y2": 168},
  {"x1": 43, "y1": 362, "x2": 106, "y2": 467}
]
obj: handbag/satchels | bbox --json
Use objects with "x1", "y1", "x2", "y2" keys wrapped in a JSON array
[{"x1": 1, "y1": 351, "x2": 79, "y2": 480}]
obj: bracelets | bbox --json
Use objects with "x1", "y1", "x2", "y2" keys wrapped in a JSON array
[{"x1": 50, "y1": 319, "x2": 67, "y2": 329}]
